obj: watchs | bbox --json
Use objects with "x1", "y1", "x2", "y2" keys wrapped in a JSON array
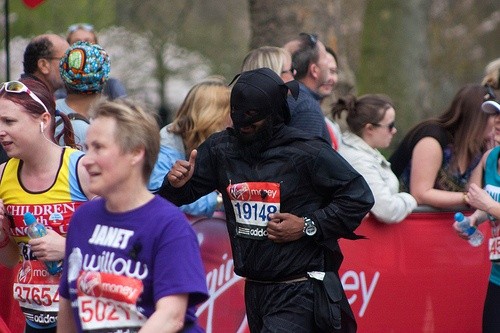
[{"x1": 302, "y1": 217, "x2": 317, "y2": 237}]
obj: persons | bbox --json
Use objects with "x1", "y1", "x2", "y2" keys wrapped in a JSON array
[
  {"x1": 0, "y1": 79, "x2": 102, "y2": 333},
  {"x1": 53, "y1": 23, "x2": 124, "y2": 99},
  {"x1": 54, "y1": 41, "x2": 111, "y2": 154},
  {"x1": 17, "y1": 33, "x2": 71, "y2": 115},
  {"x1": 388, "y1": 57, "x2": 500, "y2": 212},
  {"x1": 57, "y1": 98, "x2": 209, "y2": 333},
  {"x1": 160, "y1": 67, "x2": 375, "y2": 333},
  {"x1": 329, "y1": 93, "x2": 418, "y2": 224},
  {"x1": 147, "y1": 76, "x2": 233, "y2": 218},
  {"x1": 453, "y1": 100, "x2": 500, "y2": 333},
  {"x1": 241, "y1": 34, "x2": 342, "y2": 154}
]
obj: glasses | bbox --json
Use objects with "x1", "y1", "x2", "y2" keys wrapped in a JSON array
[
  {"x1": 44, "y1": 55, "x2": 64, "y2": 62},
  {"x1": 0, "y1": 80, "x2": 48, "y2": 113},
  {"x1": 68, "y1": 22, "x2": 94, "y2": 32},
  {"x1": 300, "y1": 30, "x2": 318, "y2": 48},
  {"x1": 282, "y1": 66, "x2": 295, "y2": 75},
  {"x1": 371, "y1": 121, "x2": 394, "y2": 132},
  {"x1": 483, "y1": 86, "x2": 495, "y2": 100}
]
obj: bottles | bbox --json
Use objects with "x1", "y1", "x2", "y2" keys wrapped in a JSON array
[
  {"x1": 454, "y1": 211, "x2": 485, "y2": 247},
  {"x1": 24, "y1": 212, "x2": 65, "y2": 275}
]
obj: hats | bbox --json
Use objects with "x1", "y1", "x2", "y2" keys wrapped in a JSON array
[
  {"x1": 229, "y1": 67, "x2": 291, "y2": 126},
  {"x1": 482, "y1": 95, "x2": 500, "y2": 113},
  {"x1": 60, "y1": 40, "x2": 111, "y2": 94}
]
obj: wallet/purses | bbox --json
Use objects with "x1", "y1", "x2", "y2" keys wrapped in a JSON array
[{"x1": 311, "y1": 274, "x2": 342, "y2": 331}]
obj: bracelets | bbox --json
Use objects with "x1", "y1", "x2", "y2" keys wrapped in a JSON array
[{"x1": 0, "y1": 236, "x2": 10, "y2": 248}]
